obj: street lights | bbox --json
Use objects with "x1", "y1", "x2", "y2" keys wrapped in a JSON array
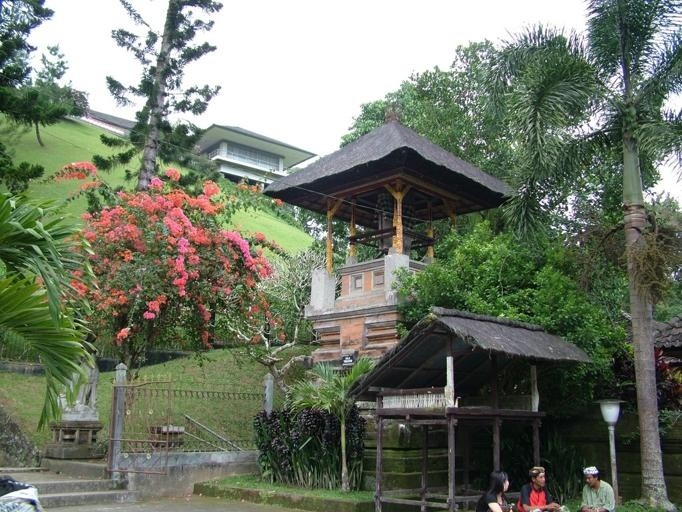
[{"x1": 591, "y1": 396, "x2": 625, "y2": 508}]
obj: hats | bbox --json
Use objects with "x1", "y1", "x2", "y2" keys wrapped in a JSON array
[{"x1": 584, "y1": 466, "x2": 599, "y2": 476}]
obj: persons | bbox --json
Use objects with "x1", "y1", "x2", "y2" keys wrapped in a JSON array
[
  {"x1": 476, "y1": 470, "x2": 514, "y2": 512},
  {"x1": 516, "y1": 466, "x2": 560, "y2": 512},
  {"x1": 576, "y1": 466, "x2": 615, "y2": 512}
]
